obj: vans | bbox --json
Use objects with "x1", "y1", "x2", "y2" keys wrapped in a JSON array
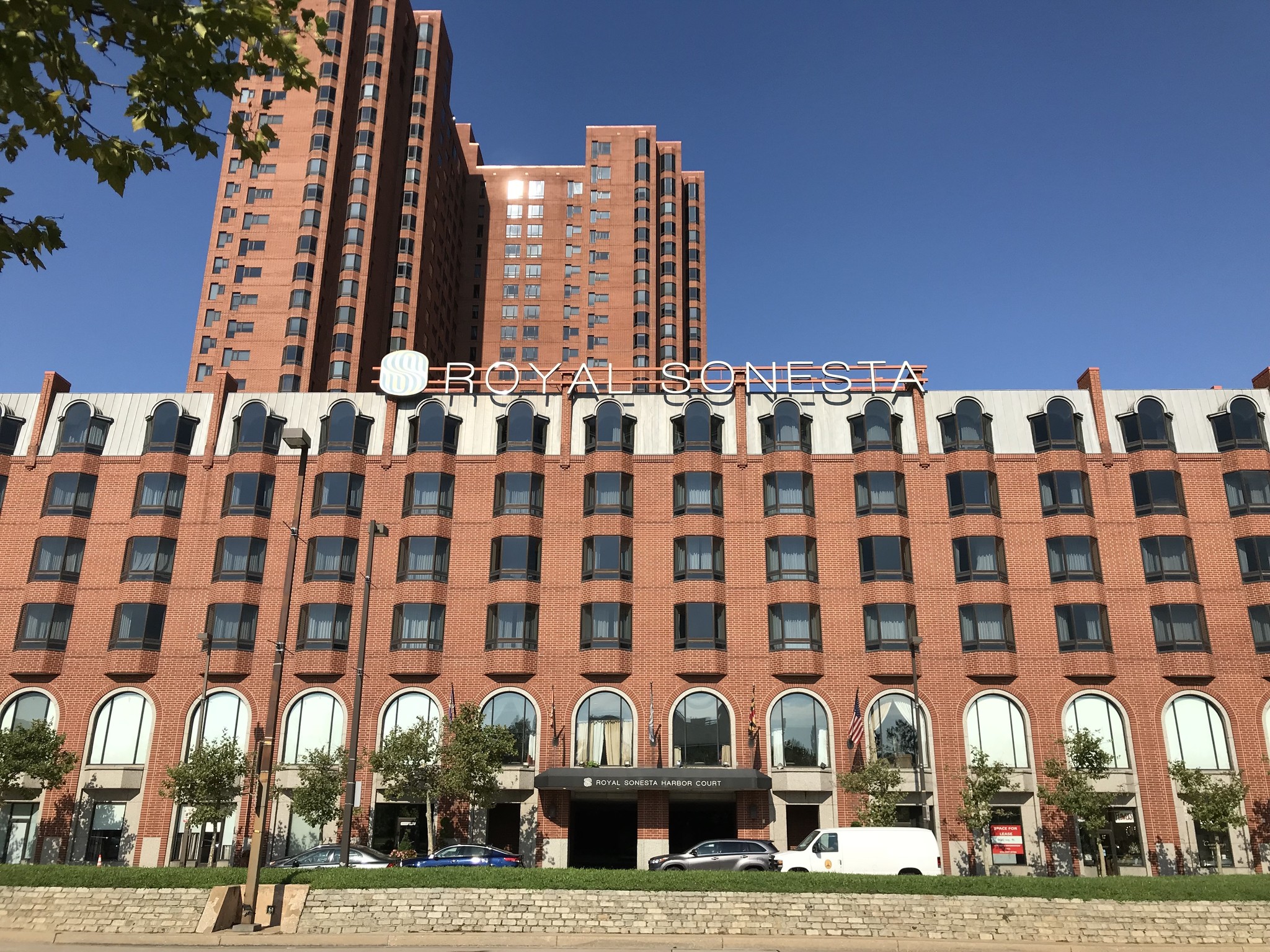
[{"x1": 768, "y1": 827, "x2": 942, "y2": 872}]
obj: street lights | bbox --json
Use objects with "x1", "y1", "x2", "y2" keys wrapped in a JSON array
[
  {"x1": 909, "y1": 635, "x2": 928, "y2": 830},
  {"x1": 336, "y1": 521, "x2": 390, "y2": 865},
  {"x1": 230, "y1": 426, "x2": 312, "y2": 934},
  {"x1": 180, "y1": 633, "x2": 215, "y2": 866}
]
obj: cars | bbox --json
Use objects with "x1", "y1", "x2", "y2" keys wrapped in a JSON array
[
  {"x1": 262, "y1": 843, "x2": 402, "y2": 869},
  {"x1": 401, "y1": 843, "x2": 526, "y2": 867}
]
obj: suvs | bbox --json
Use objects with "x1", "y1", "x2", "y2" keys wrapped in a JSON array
[{"x1": 647, "y1": 838, "x2": 780, "y2": 872}]
